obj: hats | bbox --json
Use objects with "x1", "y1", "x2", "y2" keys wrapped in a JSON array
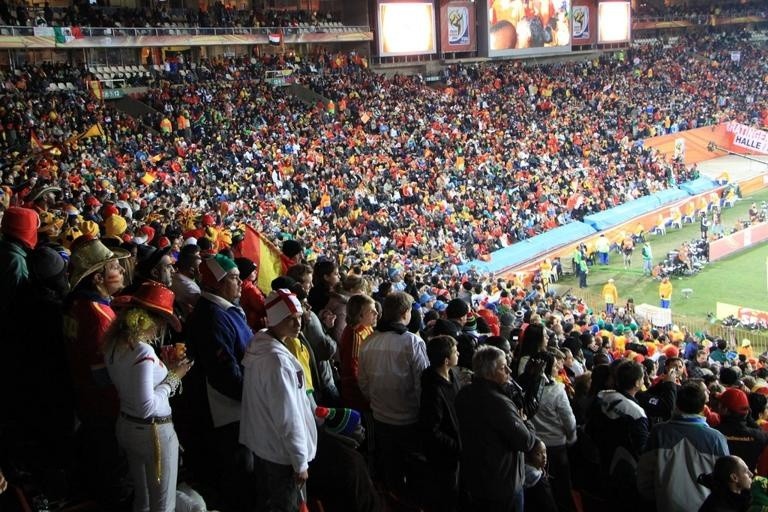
[
  {"x1": 198, "y1": 253, "x2": 237, "y2": 288},
  {"x1": 2, "y1": 206, "x2": 41, "y2": 250},
  {"x1": 85, "y1": 196, "x2": 103, "y2": 208},
  {"x1": 82, "y1": 219, "x2": 100, "y2": 236},
  {"x1": 235, "y1": 256, "x2": 258, "y2": 279},
  {"x1": 283, "y1": 239, "x2": 303, "y2": 259},
  {"x1": 104, "y1": 213, "x2": 128, "y2": 235},
  {"x1": 63, "y1": 233, "x2": 131, "y2": 289},
  {"x1": 136, "y1": 243, "x2": 172, "y2": 274},
  {"x1": 714, "y1": 387, "x2": 751, "y2": 415},
  {"x1": 29, "y1": 181, "x2": 62, "y2": 206},
  {"x1": 316, "y1": 405, "x2": 359, "y2": 434},
  {"x1": 263, "y1": 287, "x2": 304, "y2": 327},
  {"x1": 445, "y1": 297, "x2": 469, "y2": 318},
  {"x1": 112, "y1": 281, "x2": 183, "y2": 333}
]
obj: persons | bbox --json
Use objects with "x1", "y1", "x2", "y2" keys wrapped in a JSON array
[
  {"x1": 285, "y1": 261, "x2": 343, "y2": 401},
  {"x1": 684, "y1": 198, "x2": 697, "y2": 224},
  {"x1": 599, "y1": 275, "x2": 620, "y2": 314},
  {"x1": 586, "y1": 191, "x2": 650, "y2": 215},
  {"x1": 577, "y1": 257, "x2": 592, "y2": 288},
  {"x1": 523, "y1": 275, "x2": 605, "y2": 350},
  {"x1": 659, "y1": 273, "x2": 673, "y2": 311},
  {"x1": 525, "y1": 351, "x2": 581, "y2": 512},
  {"x1": 642, "y1": 236, "x2": 654, "y2": 274},
  {"x1": 356, "y1": 289, "x2": 430, "y2": 428},
  {"x1": 632, "y1": 381, "x2": 733, "y2": 509},
  {"x1": 2, "y1": 49, "x2": 539, "y2": 187},
  {"x1": 196, "y1": 253, "x2": 256, "y2": 499},
  {"x1": 540, "y1": 152, "x2": 700, "y2": 191},
  {"x1": 596, "y1": 358, "x2": 652, "y2": 465},
  {"x1": 404, "y1": 187, "x2": 538, "y2": 333},
  {"x1": 524, "y1": 434, "x2": 560, "y2": 511},
  {"x1": 0, "y1": 1, "x2": 338, "y2": 38},
  {"x1": 50, "y1": 239, "x2": 130, "y2": 491},
  {"x1": 417, "y1": 332, "x2": 461, "y2": 479},
  {"x1": 306, "y1": 235, "x2": 411, "y2": 271},
  {"x1": 665, "y1": 205, "x2": 682, "y2": 232},
  {"x1": 650, "y1": 211, "x2": 664, "y2": 234},
  {"x1": 326, "y1": 270, "x2": 369, "y2": 344},
  {"x1": 710, "y1": 198, "x2": 768, "y2": 233},
  {"x1": 747, "y1": 389, "x2": 768, "y2": 432},
  {"x1": 695, "y1": 454, "x2": 756, "y2": 512},
  {"x1": 270, "y1": 275, "x2": 367, "y2": 448},
  {"x1": 619, "y1": 231, "x2": 634, "y2": 268},
  {"x1": 609, "y1": 227, "x2": 623, "y2": 250},
  {"x1": 699, "y1": 197, "x2": 710, "y2": 219},
  {"x1": 454, "y1": 344, "x2": 539, "y2": 510},
  {"x1": 713, "y1": 388, "x2": 766, "y2": 458},
  {"x1": 517, "y1": 322, "x2": 551, "y2": 391},
  {"x1": 308, "y1": 257, "x2": 342, "y2": 310},
  {"x1": 636, "y1": 0, "x2": 768, "y2": 24},
  {"x1": 711, "y1": 192, "x2": 723, "y2": 212},
  {"x1": 6, "y1": 187, "x2": 407, "y2": 238},
  {"x1": 540, "y1": 254, "x2": 563, "y2": 283},
  {"x1": 538, "y1": 191, "x2": 586, "y2": 234},
  {"x1": 540, "y1": 32, "x2": 768, "y2": 149},
  {"x1": 723, "y1": 183, "x2": 735, "y2": 207},
  {"x1": 100, "y1": 274, "x2": 197, "y2": 512},
  {"x1": 280, "y1": 239, "x2": 307, "y2": 266},
  {"x1": 130, "y1": 238, "x2": 197, "y2": 272},
  {"x1": 596, "y1": 296, "x2": 768, "y2": 382},
  {"x1": 631, "y1": 221, "x2": 647, "y2": 244},
  {"x1": 477, "y1": 334, "x2": 548, "y2": 422},
  {"x1": 595, "y1": 232, "x2": 610, "y2": 267},
  {"x1": 340, "y1": 293, "x2": 381, "y2": 405},
  {"x1": 573, "y1": 246, "x2": 583, "y2": 276},
  {"x1": 657, "y1": 233, "x2": 732, "y2": 281},
  {"x1": 580, "y1": 243, "x2": 589, "y2": 264},
  {"x1": 700, "y1": 211, "x2": 710, "y2": 240},
  {"x1": 235, "y1": 288, "x2": 320, "y2": 507}
]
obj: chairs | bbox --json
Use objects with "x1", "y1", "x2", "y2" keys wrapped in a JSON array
[
  {"x1": 549, "y1": 454, "x2": 577, "y2": 511},
  {"x1": 10, "y1": 484, "x2": 131, "y2": 510},
  {"x1": 317, "y1": 429, "x2": 381, "y2": 506}
]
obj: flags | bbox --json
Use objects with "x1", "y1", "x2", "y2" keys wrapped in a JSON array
[
  {"x1": 31, "y1": 25, "x2": 84, "y2": 48},
  {"x1": 239, "y1": 223, "x2": 296, "y2": 296},
  {"x1": 267, "y1": 33, "x2": 282, "y2": 44}
]
